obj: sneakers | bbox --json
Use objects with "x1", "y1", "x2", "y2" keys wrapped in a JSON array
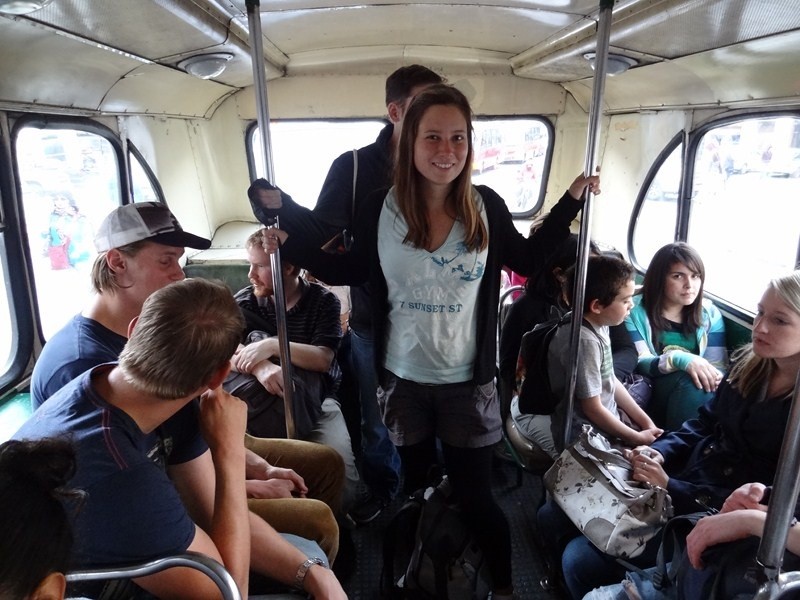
[{"x1": 351, "y1": 482, "x2": 393, "y2": 523}]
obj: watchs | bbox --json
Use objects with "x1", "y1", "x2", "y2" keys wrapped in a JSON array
[{"x1": 291, "y1": 557, "x2": 329, "y2": 594}]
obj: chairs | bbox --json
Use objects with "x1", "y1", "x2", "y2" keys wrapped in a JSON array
[{"x1": 499, "y1": 284, "x2": 555, "y2": 506}]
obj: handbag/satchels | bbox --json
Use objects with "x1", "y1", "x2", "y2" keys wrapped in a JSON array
[{"x1": 542, "y1": 424, "x2": 675, "y2": 559}]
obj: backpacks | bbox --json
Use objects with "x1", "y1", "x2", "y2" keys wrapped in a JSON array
[
  {"x1": 378, "y1": 465, "x2": 490, "y2": 600},
  {"x1": 515, "y1": 305, "x2": 604, "y2": 415},
  {"x1": 222, "y1": 303, "x2": 328, "y2": 439},
  {"x1": 616, "y1": 486, "x2": 774, "y2": 600}
]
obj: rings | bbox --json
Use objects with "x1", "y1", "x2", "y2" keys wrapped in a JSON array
[{"x1": 643, "y1": 462, "x2": 646, "y2": 470}]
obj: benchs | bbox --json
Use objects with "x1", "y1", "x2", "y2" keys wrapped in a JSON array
[{"x1": 182, "y1": 264, "x2": 255, "y2": 293}]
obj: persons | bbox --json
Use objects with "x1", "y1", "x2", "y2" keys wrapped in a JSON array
[
  {"x1": 623, "y1": 242, "x2": 730, "y2": 442},
  {"x1": 535, "y1": 268, "x2": 799, "y2": 600},
  {"x1": 510, "y1": 212, "x2": 572, "y2": 303},
  {"x1": 221, "y1": 226, "x2": 364, "y2": 517},
  {"x1": 301, "y1": 268, "x2": 353, "y2": 337},
  {"x1": 29, "y1": 200, "x2": 346, "y2": 573},
  {"x1": 0, "y1": 432, "x2": 92, "y2": 600},
  {"x1": 245, "y1": 63, "x2": 452, "y2": 528},
  {"x1": 581, "y1": 481, "x2": 799, "y2": 599},
  {"x1": 543, "y1": 252, "x2": 666, "y2": 458},
  {"x1": 262, "y1": 83, "x2": 603, "y2": 600},
  {"x1": 496, "y1": 232, "x2": 639, "y2": 469},
  {"x1": 9, "y1": 277, "x2": 352, "y2": 600},
  {"x1": 43, "y1": 195, "x2": 88, "y2": 272}
]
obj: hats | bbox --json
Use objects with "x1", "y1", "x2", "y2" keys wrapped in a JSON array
[{"x1": 95, "y1": 201, "x2": 212, "y2": 253}]
